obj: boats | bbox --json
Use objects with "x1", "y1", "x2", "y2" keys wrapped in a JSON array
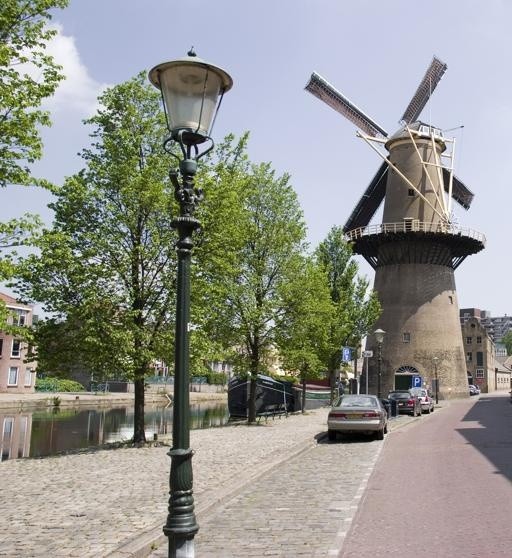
[{"x1": 228, "y1": 371, "x2": 303, "y2": 418}]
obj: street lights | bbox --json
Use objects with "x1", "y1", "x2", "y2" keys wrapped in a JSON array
[
  {"x1": 149, "y1": 46, "x2": 233, "y2": 558},
  {"x1": 374, "y1": 329, "x2": 386, "y2": 400},
  {"x1": 433, "y1": 357, "x2": 439, "y2": 404}
]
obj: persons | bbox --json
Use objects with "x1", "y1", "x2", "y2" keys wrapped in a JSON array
[{"x1": 338, "y1": 377, "x2": 346, "y2": 396}]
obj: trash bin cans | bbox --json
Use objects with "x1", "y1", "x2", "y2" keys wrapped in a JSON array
[{"x1": 382, "y1": 398, "x2": 390, "y2": 419}]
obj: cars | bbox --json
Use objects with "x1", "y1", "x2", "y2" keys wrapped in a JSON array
[
  {"x1": 327, "y1": 387, "x2": 434, "y2": 440},
  {"x1": 469, "y1": 385, "x2": 480, "y2": 396}
]
